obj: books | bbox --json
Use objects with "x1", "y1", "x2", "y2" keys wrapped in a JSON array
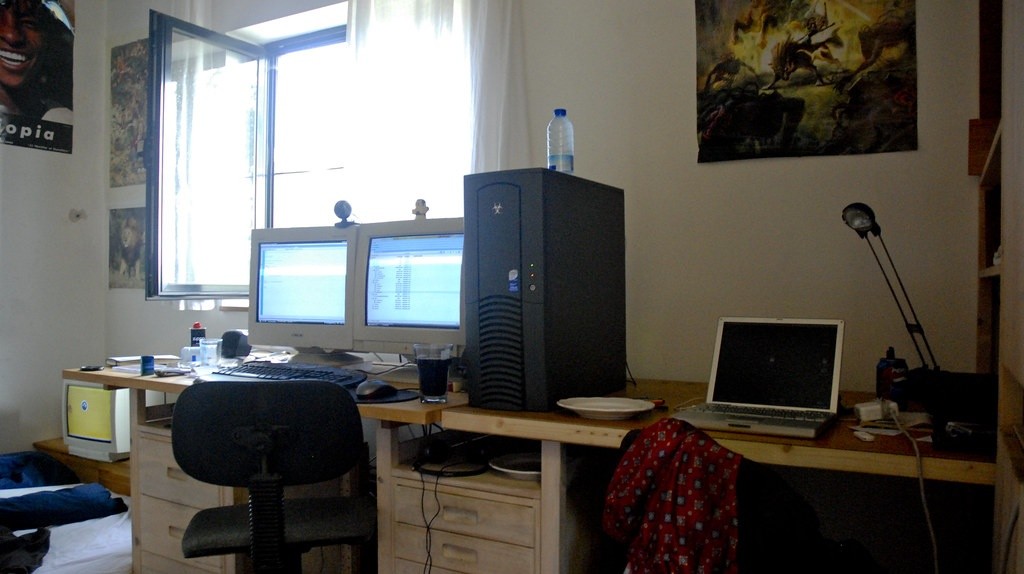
[{"x1": 105, "y1": 354, "x2": 182, "y2": 374}]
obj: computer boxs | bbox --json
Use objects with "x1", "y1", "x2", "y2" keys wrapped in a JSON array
[{"x1": 460, "y1": 168, "x2": 625, "y2": 413}]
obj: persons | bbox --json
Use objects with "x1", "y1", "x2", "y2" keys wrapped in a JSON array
[
  {"x1": 0, "y1": 0, "x2": 74, "y2": 115},
  {"x1": 40, "y1": 70, "x2": 73, "y2": 125}
]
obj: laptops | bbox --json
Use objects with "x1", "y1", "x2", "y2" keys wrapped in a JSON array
[{"x1": 672, "y1": 317, "x2": 845, "y2": 440}]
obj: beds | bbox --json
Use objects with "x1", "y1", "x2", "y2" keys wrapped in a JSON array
[{"x1": 0, "y1": 481, "x2": 133, "y2": 574}]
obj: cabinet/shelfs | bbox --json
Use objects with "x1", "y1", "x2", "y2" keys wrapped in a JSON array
[{"x1": 978, "y1": 0, "x2": 1024, "y2": 573}]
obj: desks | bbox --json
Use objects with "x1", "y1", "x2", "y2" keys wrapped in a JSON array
[
  {"x1": 33, "y1": 435, "x2": 131, "y2": 496},
  {"x1": 62, "y1": 367, "x2": 998, "y2": 574}
]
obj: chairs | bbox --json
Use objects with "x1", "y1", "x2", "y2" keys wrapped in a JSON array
[
  {"x1": 173, "y1": 380, "x2": 377, "y2": 574},
  {"x1": 600, "y1": 417, "x2": 878, "y2": 573}
]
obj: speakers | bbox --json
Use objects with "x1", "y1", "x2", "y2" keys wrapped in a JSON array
[{"x1": 220, "y1": 330, "x2": 252, "y2": 358}]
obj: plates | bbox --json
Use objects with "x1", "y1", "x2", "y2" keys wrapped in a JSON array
[
  {"x1": 489, "y1": 452, "x2": 541, "y2": 480},
  {"x1": 556, "y1": 397, "x2": 655, "y2": 421}
]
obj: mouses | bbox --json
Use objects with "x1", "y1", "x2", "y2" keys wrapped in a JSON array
[{"x1": 355, "y1": 380, "x2": 398, "y2": 399}]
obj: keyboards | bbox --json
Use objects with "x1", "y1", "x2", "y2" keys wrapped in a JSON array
[{"x1": 197, "y1": 363, "x2": 368, "y2": 388}]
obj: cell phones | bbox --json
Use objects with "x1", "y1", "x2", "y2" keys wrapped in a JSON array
[{"x1": 81, "y1": 365, "x2": 104, "y2": 371}]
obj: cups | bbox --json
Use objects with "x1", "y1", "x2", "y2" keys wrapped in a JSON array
[
  {"x1": 200, "y1": 338, "x2": 223, "y2": 369},
  {"x1": 140, "y1": 355, "x2": 154, "y2": 375},
  {"x1": 414, "y1": 343, "x2": 454, "y2": 404}
]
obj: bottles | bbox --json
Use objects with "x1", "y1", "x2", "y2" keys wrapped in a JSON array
[
  {"x1": 546, "y1": 108, "x2": 574, "y2": 177},
  {"x1": 189, "y1": 322, "x2": 206, "y2": 347}
]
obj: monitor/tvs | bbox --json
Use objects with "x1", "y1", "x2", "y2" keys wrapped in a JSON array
[
  {"x1": 247, "y1": 218, "x2": 466, "y2": 384},
  {"x1": 60, "y1": 379, "x2": 133, "y2": 464}
]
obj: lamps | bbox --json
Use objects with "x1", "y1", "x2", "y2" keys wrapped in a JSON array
[{"x1": 842, "y1": 202, "x2": 943, "y2": 412}]
